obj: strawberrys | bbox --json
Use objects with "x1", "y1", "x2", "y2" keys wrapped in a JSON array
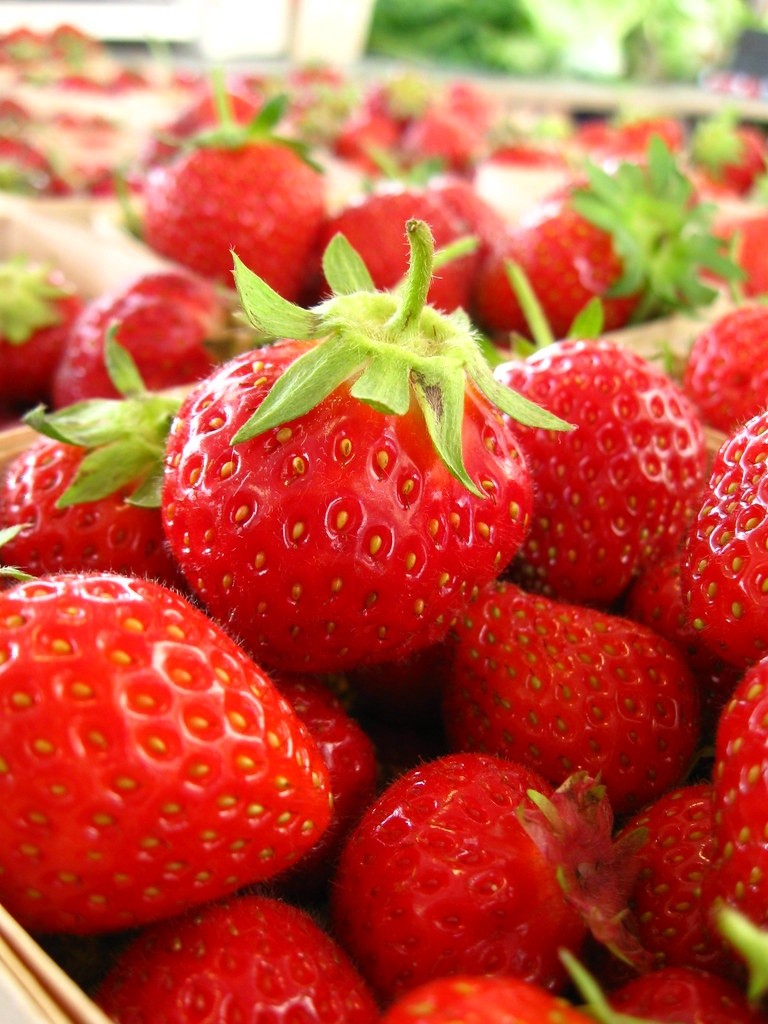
[{"x1": 0, "y1": 22, "x2": 768, "y2": 1024}]
